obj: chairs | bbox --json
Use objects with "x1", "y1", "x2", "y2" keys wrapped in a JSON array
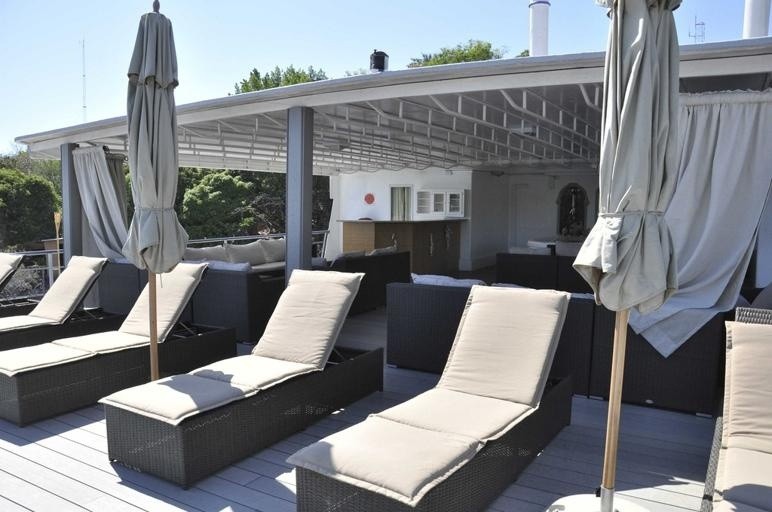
[
  {"x1": 285, "y1": 285, "x2": 574, "y2": 512},
  {"x1": 699, "y1": 307, "x2": 771, "y2": 512},
  {"x1": 99, "y1": 269, "x2": 384, "y2": 489}
]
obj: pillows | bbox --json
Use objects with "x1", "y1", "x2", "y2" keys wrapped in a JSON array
[{"x1": 411, "y1": 273, "x2": 489, "y2": 288}]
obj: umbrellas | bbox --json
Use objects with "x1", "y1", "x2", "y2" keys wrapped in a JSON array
[
  {"x1": 122, "y1": 0, "x2": 190, "y2": 382},
  {"x1": 573, "y1": 0, "x2": 692, "y2": 512}
]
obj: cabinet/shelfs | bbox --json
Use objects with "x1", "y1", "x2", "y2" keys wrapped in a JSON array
[
  {"x1": 337, "y1": 218, "x2": 469, "y2": 274},
  {"x1": 414, "y1": 189, "x2": 465, "y2": 219}
]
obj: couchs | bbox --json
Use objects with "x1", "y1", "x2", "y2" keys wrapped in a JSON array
[
  {"x1": 589, "y1": 284, "x2": 771, "y2": 418},
  {"x1": 385, "y1": 272, "x2": 594, "y2": 398},
  {"x1": 495, "y1": 240, "x2": 594, "y2": 294}
]
